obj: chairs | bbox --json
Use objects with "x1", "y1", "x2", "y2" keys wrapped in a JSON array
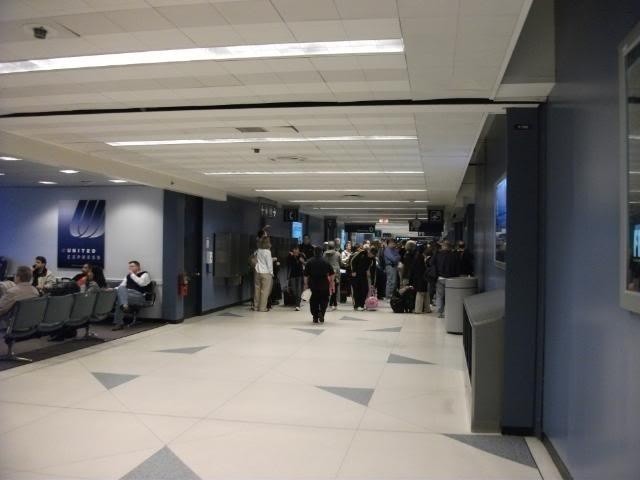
[{"x1": 2, "y1": 278, "x2": 158, "y2": 367}]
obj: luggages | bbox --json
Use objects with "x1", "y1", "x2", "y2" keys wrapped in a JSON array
[
  {"x1": 284, "y1": 286, "x2": 295, "y2": 305},
  {"x1": 365, "y1": 287, "x2": 378, "y2": 311}
]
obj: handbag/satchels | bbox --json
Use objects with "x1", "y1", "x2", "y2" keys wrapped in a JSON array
[{"x1": 391, "y1": 285, "x2": 414, "y2": 314}]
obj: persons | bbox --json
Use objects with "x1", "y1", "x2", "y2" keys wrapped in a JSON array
[
  {"x1": 31, "y1": 256, "x2": 48, "y2": 287},
  {"x1": 46, "y1": 265, "x2": 107, "y2": 341},
  {"x1": 72, "y1": 263, "x2": 91, "y2": 280},
  {"x1": 0, "y1": 265, "x2": 39, "y2": 332},
  {"x1": 247, "y1": 224, "x2": 475, "y2": 323},
  {"x1": 108, "y1": 261, "x2": 154, "y2": 331}
]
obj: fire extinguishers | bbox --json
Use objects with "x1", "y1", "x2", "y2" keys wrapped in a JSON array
[{"x1": 178, "y1": 267, "x2": 189, "y2": 295}]
